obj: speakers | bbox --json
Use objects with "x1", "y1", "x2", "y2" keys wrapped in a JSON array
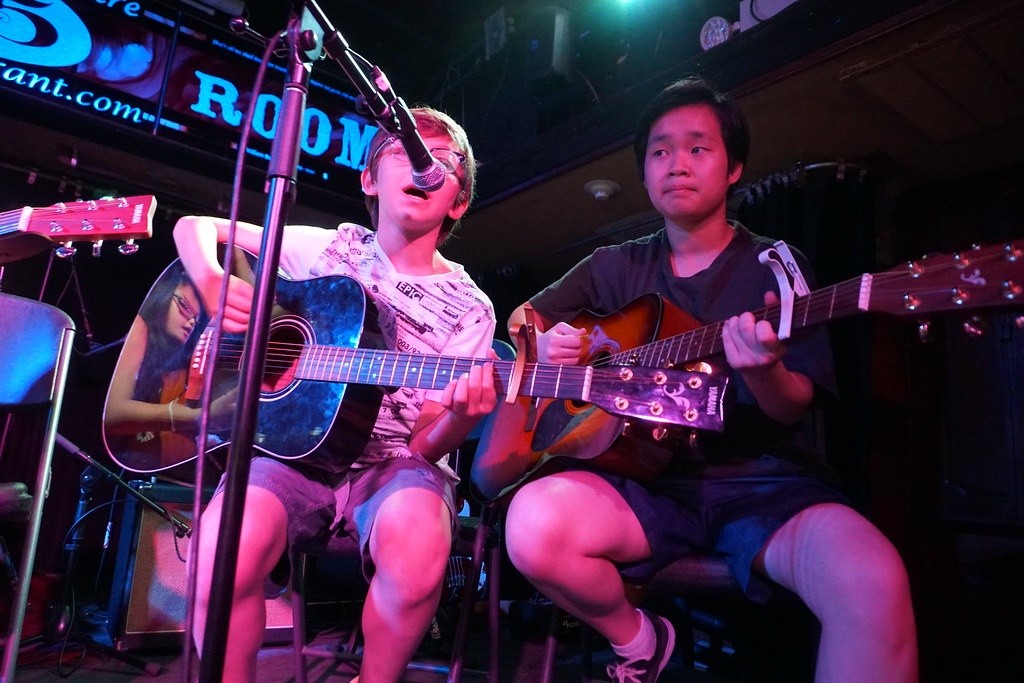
[{"x1": 109, "y1": 479, "x2": 294, "y2": 649}]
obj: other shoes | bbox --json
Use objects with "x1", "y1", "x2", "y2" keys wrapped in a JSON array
[{"x1": 606, "y1": 607, "x2": 676, "y2": 683}]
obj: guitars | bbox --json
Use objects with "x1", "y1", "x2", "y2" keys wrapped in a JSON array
[
  {"x1": 470, "y1": 241, "x2": 1022, "y2": 505},
  {"x1": 0, "y1": 194, "x2": 164, "y2": 266},
  {"x1": 101, "y1": 239, "x2": 733, "y2": 489}
]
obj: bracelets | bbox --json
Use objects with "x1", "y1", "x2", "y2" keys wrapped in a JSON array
[{"x1": 169, "y1": 402, "x2": 176, "y2": 432}]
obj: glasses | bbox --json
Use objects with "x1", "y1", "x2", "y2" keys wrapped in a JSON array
[
  {"x1": 372, "y1": 139, "x2": 468, "y2": 189},
  {"x1": 173, "y1": 294, "x2": 200, "y2": 325}
]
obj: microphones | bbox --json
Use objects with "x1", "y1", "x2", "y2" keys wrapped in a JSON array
[{"x1": 369, "y1": 64, "x2": 446, "y2": 192}]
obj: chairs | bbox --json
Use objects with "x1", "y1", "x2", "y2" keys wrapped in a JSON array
[
  {"x1": 291, "y1": 342, "x2": 515, "y2": 683},
  {"x1": 0, "y1": 296, "x2": 77, "y2": 683},
  {"x1": 543, "y1": 406, "x2": 825, "y2": 683}
]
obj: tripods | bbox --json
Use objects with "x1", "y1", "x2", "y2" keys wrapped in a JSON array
[{"x1": 0, "y1": 428, "x2": 192, "y2": 676}]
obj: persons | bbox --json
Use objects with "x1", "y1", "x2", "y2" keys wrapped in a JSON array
[
  {"x1": 172, "y1": 108, "x2": 503, "y2": 683},
  {"x1": 105, "y1": 274, "x2": 239, "y2": 471},
  {"x1": 507, "y1": 79, "x2": 917, "y2": 683}
]
